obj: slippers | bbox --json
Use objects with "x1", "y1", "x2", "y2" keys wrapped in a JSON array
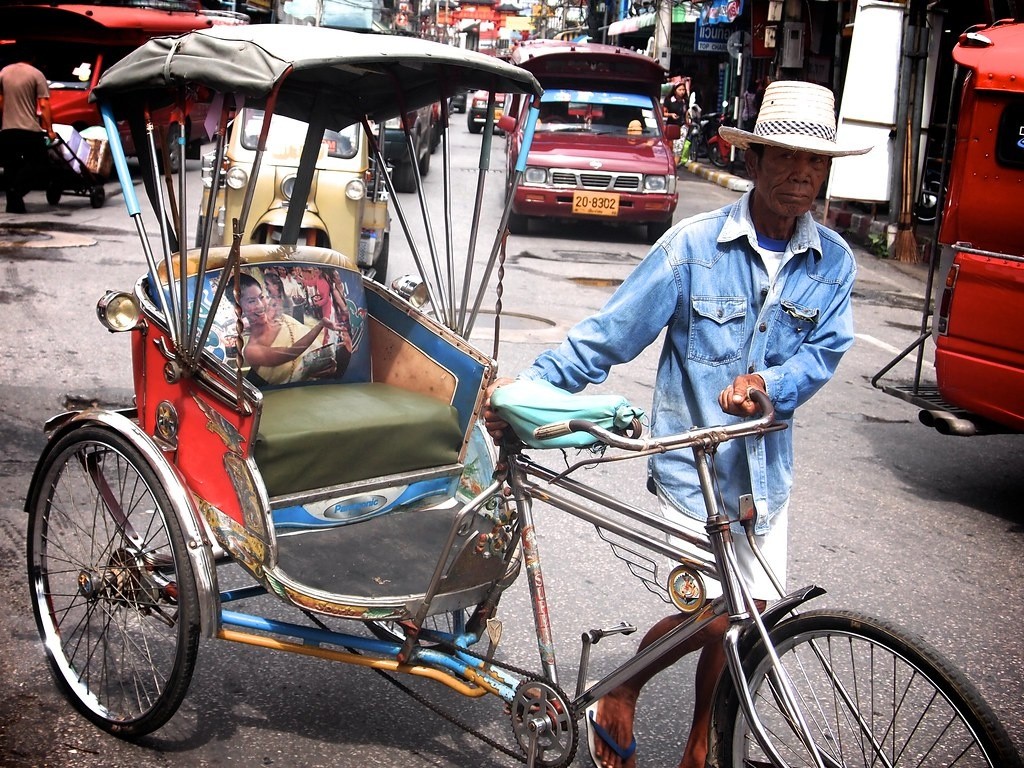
[{"x1": 582, "y1": 676, "x2": 637, "y2": 768}]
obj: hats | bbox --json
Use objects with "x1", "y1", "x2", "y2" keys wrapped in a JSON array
[{"x1": 717, "y1": 81, "x2": 873, "y2": 158}]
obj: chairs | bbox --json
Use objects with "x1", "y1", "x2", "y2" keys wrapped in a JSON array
[{"x1": 151, "y1": 236, "x2": 467, "y2": 486}]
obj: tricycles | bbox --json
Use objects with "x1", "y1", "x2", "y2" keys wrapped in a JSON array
[{"x1": 23, "y1": 18, "x2": 1022, "y2": 766}]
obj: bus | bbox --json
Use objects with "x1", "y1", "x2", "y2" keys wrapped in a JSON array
[{"x1": 865, "y1": 13, "x2": 1023, "y2": 450}]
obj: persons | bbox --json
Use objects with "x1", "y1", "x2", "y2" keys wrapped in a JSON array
[
  {"x1": 0, "y1": 48, "x2": 56, "y2": 214},
  {"x1": 226, "y1": 274, "x2": 350, "y2": 387},
  {"x1": 662, "y1": 81, "x2": 692, "y2": 180},
  {"x1": 483, "y1": 81, "x2": 878, "y2": 767}
]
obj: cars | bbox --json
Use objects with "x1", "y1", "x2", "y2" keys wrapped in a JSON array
[{"x1": 17, "y1": 3, "x2": 513, "y2": 319}]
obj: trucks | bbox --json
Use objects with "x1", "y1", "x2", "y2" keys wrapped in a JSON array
[{"x1": 496, "y1": 38, "x2": 681, "y2": 247}]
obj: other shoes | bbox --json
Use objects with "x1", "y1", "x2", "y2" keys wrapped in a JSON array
[
  {"x1": 43, "y1": 185, "x2": 65, "y2": 206},
  {"x1": 4, "y1": 201, "x2": 27, "y2": 214}
]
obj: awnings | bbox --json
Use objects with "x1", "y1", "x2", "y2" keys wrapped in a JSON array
[
  {"x1": 553, "y1": 31, "x2": 593, "y2": 44},
  {"x1": 608, "y1": 2, "x2": 703, "y2": 36}
]
